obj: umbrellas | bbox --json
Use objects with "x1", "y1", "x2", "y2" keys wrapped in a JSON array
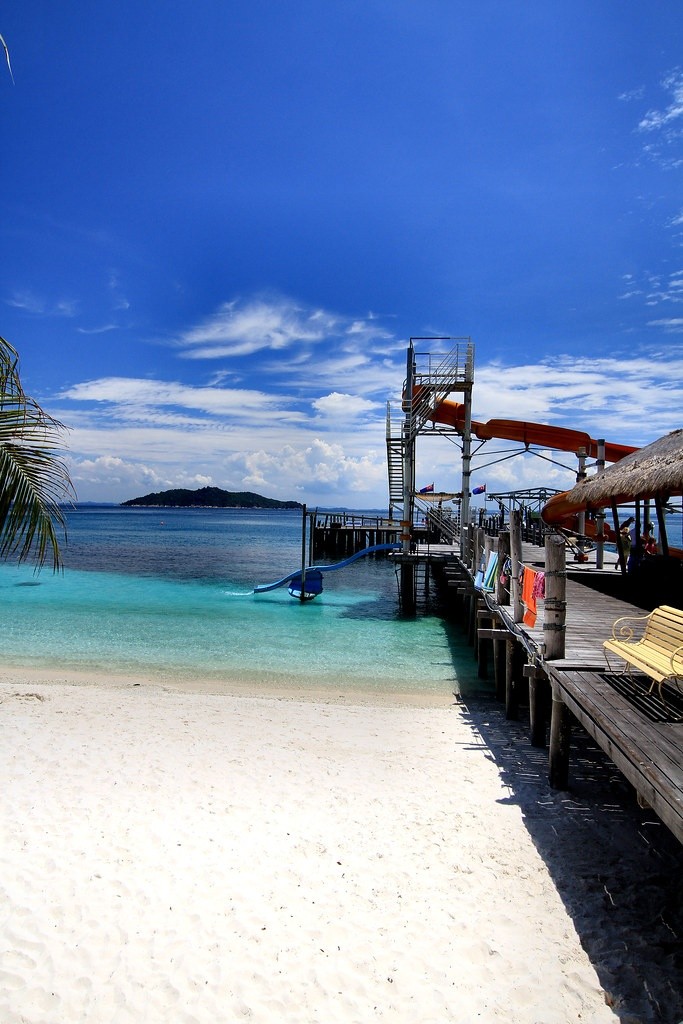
[{"x1": 565, "y1": 428, "x2": 683, "y2": 505}]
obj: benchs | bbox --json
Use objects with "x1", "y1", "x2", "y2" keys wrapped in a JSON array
[{"x1": 602, "y1": 605, "x2": 683, "y2": 720}]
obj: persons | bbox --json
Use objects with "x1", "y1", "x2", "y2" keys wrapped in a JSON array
[
  {"x1": 625, "y1": 523, "x2": 641, "y2": 571},
  {"x1": 425, "y1": 517, "x2": 429, "y2": 525},
  {"x1": 641, "y1": 524, "x2": 658, "y2": 558},
  {"x1": 615, "y1": 521, "x2": 631, "y2": 570}
]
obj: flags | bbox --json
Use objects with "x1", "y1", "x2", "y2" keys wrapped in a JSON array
[
  {"x1": 473, "y1": 485, "x2": 485, "y2": 495},
  {"x1": 420, "y1": 484, "x2": 433, "y2": 493}
]
